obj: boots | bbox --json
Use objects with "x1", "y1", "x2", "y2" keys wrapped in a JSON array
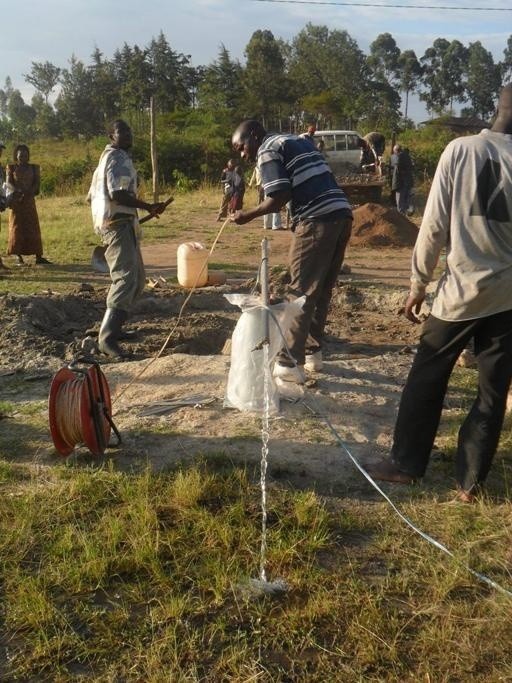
[{"x1": 97, "y1": 306, "x2": 136, "y2": 359}]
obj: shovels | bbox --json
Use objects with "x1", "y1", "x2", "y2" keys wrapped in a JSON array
[{"x1": 91, "y1": 197, "x2": 174, "y2": 272}]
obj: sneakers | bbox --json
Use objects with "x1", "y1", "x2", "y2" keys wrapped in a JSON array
[
  {"x1": 36, "y1": 258, "x2": 53, "y2": 265},
  {"x1": 263, "y1": 226, "x2": 286, "y2": 231},
  {"x1": 16, "y1": 257, "x2": 24, "y2": 265},
  {"x1": 304, "y1": 349, "x2": 324, "y2": 373},
  {"x1": 271, "y1": 362, "x2": 305, "y2": 385}
]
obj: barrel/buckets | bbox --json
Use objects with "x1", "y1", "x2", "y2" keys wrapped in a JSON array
[{"x1": 175, "y1": 241, "x2": 209, "y2": 289}]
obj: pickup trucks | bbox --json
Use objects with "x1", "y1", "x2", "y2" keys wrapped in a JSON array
[{"x1": 298, "y1": 128, "x2": 388, "y2": 202}]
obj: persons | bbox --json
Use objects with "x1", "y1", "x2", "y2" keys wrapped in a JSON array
[
  {"x1": 0, "y1": 141, "x2": 10, "y2": 271},
  {"x1": 6, "y1": 144, "x2": 52, "y2": 264},
  {"x1": 391, "y1": 144, "x2": 414, "y2": 217},
  {"x1": 364, "y1": 82, "x2": 512, "y2": 504},
  {"x1": 214, "y1": 124, "x2": 325, "y2": 230},
  {"x1": 230, "y1": 120, "x2": 353, "y2": 385},
  {"x1": 86, "y1": 118, "x2": 167, "y2": 356}
]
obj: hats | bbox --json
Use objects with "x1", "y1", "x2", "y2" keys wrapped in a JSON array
[{"x1": 307, "y1": 125, "x2": 316, "y2": 132}]
